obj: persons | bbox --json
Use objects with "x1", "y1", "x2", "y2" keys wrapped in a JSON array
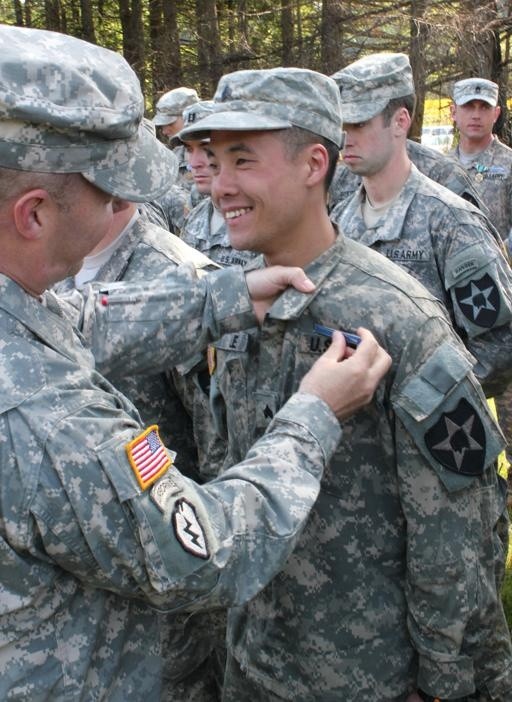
[
  {"x1": 1, "y1": 21, "x2": 393, "y2": 702},
  {"x1": 50, "y1": 88, "x2": 488, "y2": 483},
  {"x1": 331, "y1": 55, "x2": 510, "y2": 384},
  {"x1": 180, "y1": 68, "x2": 511, "y2": 702},
  {"x1": 453, "y1": 80, "x2": 511, "y2": 256}
]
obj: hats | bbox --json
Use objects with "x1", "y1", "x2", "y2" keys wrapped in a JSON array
[
  {"x1": 0, "y1": 24, "x2": 179, "y2": 202},
  {"x1": 151, "y1": 51, "x2": 415, "y2": 150},
  {"x1": 452, "y1": 78, "x2": 498, "y2": 108}
]
